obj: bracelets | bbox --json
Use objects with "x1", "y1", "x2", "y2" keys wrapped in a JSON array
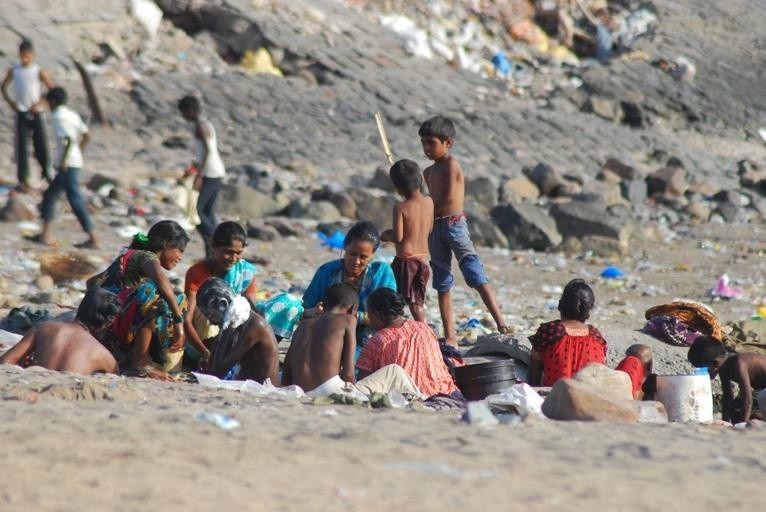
[
  {"x1": 358, "y1": 311, "x2": 362, "y2": 324},
  {"x1": 364, "y1": 311, "x2": 369, "y2": 326},
  {"x1": 355, "y1": 312, "x2": 359, "y2": 318}
]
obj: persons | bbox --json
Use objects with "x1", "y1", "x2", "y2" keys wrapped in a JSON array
[
  {"x1": 178, "y1": 93, "x2": 226, "y2": 245},
  {"x1": 380, "y1": 158, "x2": 439, "y2": 326},
  {"x1": 25, "y1": 85, "x2": 103, "y2": 252},
  {"x1": 0, "y1": 34, "x2": 59, "y2": 193},
  {"x1": 280, "y1": 282, "x2": 465, "y2": 410},
  {"x1": 301, "y1": 221, "x2": 397, "y2": 380},
  {"x1": 85, "y1": 220, "x2": 280, "y2": 387},
  {"x1": 0, "y1": 287, "x2": 121, "y2": 377},
  {"x1": 410, "y1": 110, "x2": 516, "y2": 347},
  {"x1": 687, "y1": 330, "x2": 766, "y2": 429},
  {"x1": 525, "y1": 277, "x2": 653, "y2": 401}
]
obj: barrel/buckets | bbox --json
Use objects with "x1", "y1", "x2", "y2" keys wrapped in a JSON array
[
  {"x1": 453, "y1": 357, "x2": 517, "y2": 400},
  {"x1": 654, "y1": 367, "x2": 712, "y2": 423}
]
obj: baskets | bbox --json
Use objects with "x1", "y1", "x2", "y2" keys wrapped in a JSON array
[{"x1": 38, "y1": 254, "x2": 97, "y2": 287}]
